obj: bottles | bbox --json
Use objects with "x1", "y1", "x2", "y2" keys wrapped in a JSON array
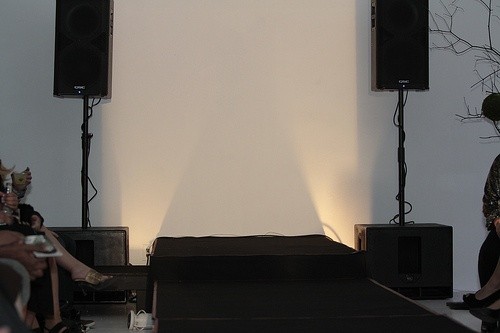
[{"x1": 2, "y1": 182, "x2": 13, "y2": 214}]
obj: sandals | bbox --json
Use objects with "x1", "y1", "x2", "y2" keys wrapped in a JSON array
[{"x1": 41, "y1": 322, "x2": 74, "y2": 333}]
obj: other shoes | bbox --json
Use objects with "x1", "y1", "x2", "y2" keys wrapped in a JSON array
[
  {"x1": 463, "y1": 288, "x2": 500, "y2": 309},
  {"x1": 62, "y1": 317, "x2": 91, "y2": 332},
  {"x1": 62, "y1": 307, "x2": 96, "y2": 326},
  {"x1": 469, "y1": 307, "x2": 500, "y2": 322}
]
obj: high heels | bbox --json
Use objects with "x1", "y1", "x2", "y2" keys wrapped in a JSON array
[{"x1": 73, "y1": 268, "x2": 116, "y2": 297}]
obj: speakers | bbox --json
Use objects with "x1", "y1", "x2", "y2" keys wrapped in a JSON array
[
  {"x1": 54, "y1": 0, "x2": 114, "y2": 98},
  {"x1": 46, "y1": 226, "x2": 128, "y2": 304},
  {"x1": 353, "y1": 223, "x2": 453, "y2": 300},
  {"x1": 371, "y1": 0, "x2": 430, "y2": 92}
]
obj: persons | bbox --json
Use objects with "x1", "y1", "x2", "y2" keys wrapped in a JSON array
[
  {"x1": 0, "y1": 168, "x2": 115, "y2": 333},
  {"x1": 463, "y1": 154, "x2": 500, "y2": 312}
]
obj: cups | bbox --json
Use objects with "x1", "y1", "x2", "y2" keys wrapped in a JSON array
[{"x1": 12, "y1": 172, "x2": 28, "y2": 190}]
